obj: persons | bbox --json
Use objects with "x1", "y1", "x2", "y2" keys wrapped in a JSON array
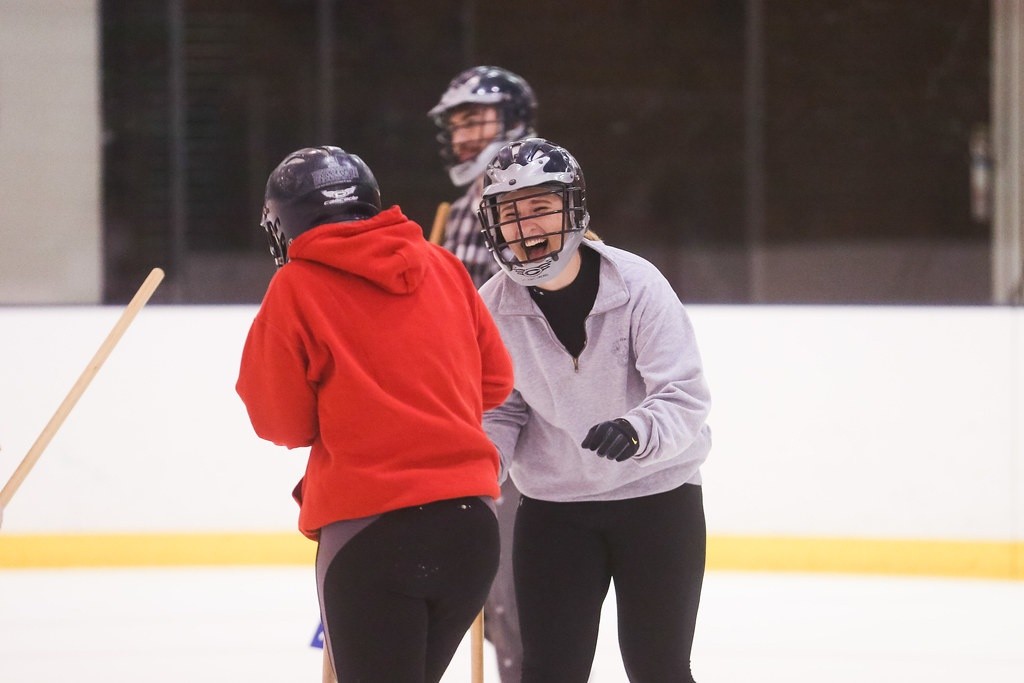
[
  {"x1": 476, "y1": 138, "x2": 712, "y2": 682},
  {"x1": 426, "y1": 64, "x2": 546, "y2": 682},
  {"x1": 235, "y1": 146, "x2": 515, "y2": 683}
]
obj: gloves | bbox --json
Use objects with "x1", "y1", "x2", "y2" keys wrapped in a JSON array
[{"x1": 581, "y1": 418, "x2": 639, "y2": 462}]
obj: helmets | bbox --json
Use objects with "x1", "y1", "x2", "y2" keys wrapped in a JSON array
[
  {"x1": 426, "y1": 65, "x2": 539, "y2": 187},
  {"x1": 477, "y1": 137, "x2": 591, "y2": 287},
  {"x1": 260, "y1": 145, "x2": 382, "y2": 268}
]
